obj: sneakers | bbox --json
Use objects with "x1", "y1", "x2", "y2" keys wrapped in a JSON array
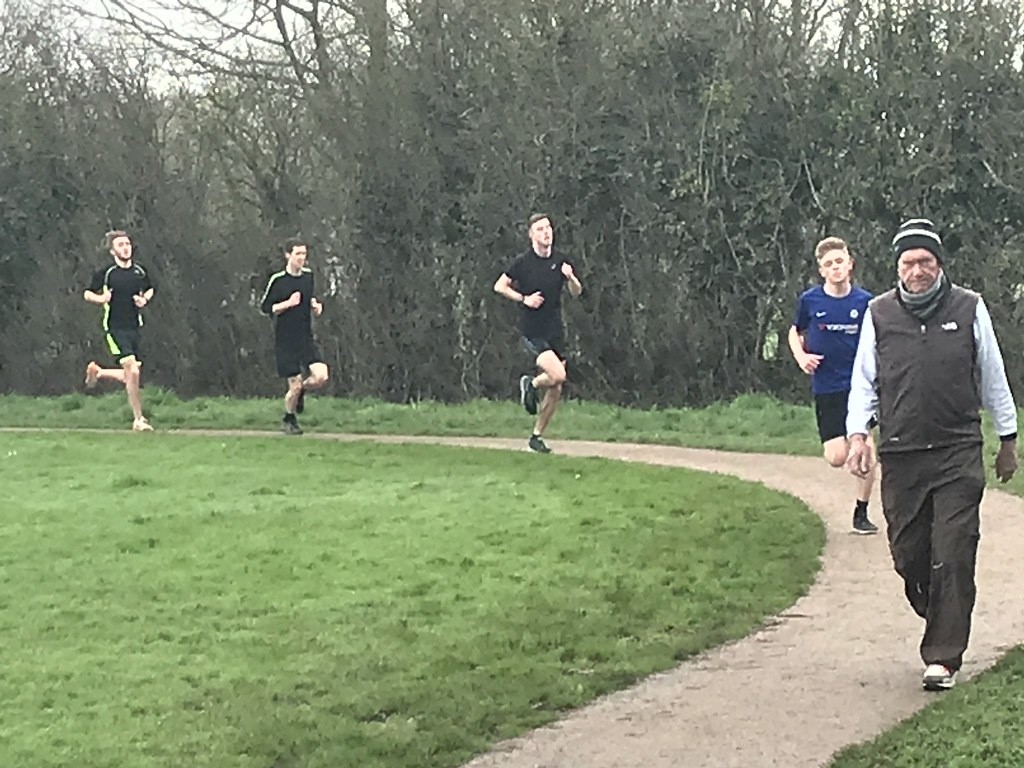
[
  {"x1": 283, "y1": 417, "x2": 303, "y2": 435},
  {"x1": 296, "y1": 390, "x2": 304, "y2": 414},
  {"x1": 520, "y1": 375, "x2": 540, "y2": 415},
  {"x1": 85, "y1": 362, "x2": 100, "y2": 388},
  {"x1": 132, "y1": 419, "x2": 154, "y2": 432},
  {"x1": 852, "y1": 515, "x2": 878, "y2": 535},
  {"x1": 922, "y1": 663, "x2": 959, "y2": 690},
  {"x1": 528, "y1": 434, "x2": 552, "y2": 454}
]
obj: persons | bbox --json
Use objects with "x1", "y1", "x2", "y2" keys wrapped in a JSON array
[
  {"x1": 260, "y1": 238, "x2": 329, "y2": 434},
  {"x1": 788, "y1": 236, "x2": 877, "y2": 533},
  {"x1": 494, "y1": 212, "x2": 582, "y2": 453},
  {"x1": 84, "y1": 229, "x2": 156, "y2": 431},
  {"x1": 846, "y1": 217, "x2": 1016, "y2": 689}
]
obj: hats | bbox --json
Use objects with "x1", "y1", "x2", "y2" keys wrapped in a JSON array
[{"x1": 893, "y1": 218, "x2": 945, "y2": 271}]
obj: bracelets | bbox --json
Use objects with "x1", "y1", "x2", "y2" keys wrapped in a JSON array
[{"x1": 519, "y1": 295, "x2": 526, "y2": 304}]
